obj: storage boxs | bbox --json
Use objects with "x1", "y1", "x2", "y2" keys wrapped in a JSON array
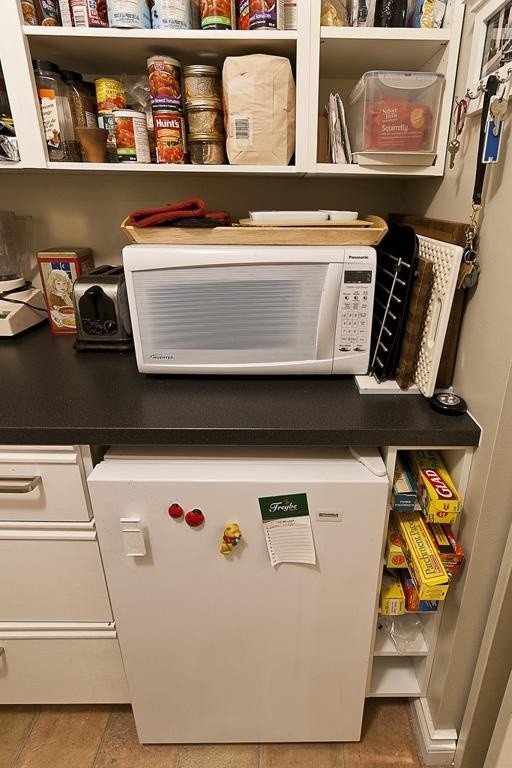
[{"x1": 344, "y1": 71, "x2": 445, "y2": 152}]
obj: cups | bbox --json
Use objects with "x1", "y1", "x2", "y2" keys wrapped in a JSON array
[{"x1": 74, "y1": 128, "x2": 109, "y2": 163}]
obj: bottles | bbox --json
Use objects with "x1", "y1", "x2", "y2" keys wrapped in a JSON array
[{"x1": 183, "y1": 63, "x2": 226, "y2": 165}]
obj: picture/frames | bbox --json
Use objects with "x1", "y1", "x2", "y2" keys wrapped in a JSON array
[{"x1": 466, "y1": 0, "x2": 512, "y2": 117}]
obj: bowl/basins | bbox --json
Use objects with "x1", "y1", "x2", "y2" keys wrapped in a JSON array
[{"x1": 318, "y1": 209, "x2": 359, "y2": 221}]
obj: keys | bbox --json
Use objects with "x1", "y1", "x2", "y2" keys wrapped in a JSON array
[
  {"x1": 455, "y1": 257, "x2": 474, "y2": 291},
  {"x1": 447, "y1": 136, "x2": 460, "y2": 170},
  {"x1": 489, "y1": 96, "x2": 508, "y2": 137}
]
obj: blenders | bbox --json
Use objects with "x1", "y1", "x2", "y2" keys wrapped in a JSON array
[{"x1": 0, "y1": 210, "x2": 50, "y2": 340}]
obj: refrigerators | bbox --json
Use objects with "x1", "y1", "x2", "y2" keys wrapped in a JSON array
[{"x1": 86, "y1": 444, "x2": 390, "y2": 744}]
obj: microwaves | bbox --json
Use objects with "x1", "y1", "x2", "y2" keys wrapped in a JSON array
[{"x1": 122, "y1": 243, "x2": 377, "y2": 375}]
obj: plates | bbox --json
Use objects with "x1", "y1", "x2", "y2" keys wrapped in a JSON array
[{"x1": 248, "y1": 210, "x2": 328, "y2": 221}]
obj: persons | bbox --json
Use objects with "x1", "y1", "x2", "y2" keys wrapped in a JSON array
[{"x1": 47, "y1": 268, "x2": 74, "y2": 311}]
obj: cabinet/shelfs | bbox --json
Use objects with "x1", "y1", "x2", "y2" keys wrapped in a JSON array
[
  {"x1": 363, "y1": 445, "x2": 473, "y2": 697},
  {"x1": 1, "y1": 1, "x2": 466, "y2": 177},
  {"x1": 0, "y1": 444, "x2": 133, "y2": 705}
]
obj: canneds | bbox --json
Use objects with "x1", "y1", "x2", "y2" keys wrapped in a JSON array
[
  {"x1": 21, "y1": 0, "x2": 297, "y2": 30},
  {"x1": 94, "y1": 54, "x2": 225, "y2": 165}
]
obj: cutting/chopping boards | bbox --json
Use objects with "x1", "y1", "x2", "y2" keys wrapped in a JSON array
[{"x1": 386, "y1": 212, "x2": 476, "y2": 398}]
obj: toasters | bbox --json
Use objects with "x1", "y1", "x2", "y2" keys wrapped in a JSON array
[{"x1": 72, "y1": 265, "x2": 135, "y2": 354}]
